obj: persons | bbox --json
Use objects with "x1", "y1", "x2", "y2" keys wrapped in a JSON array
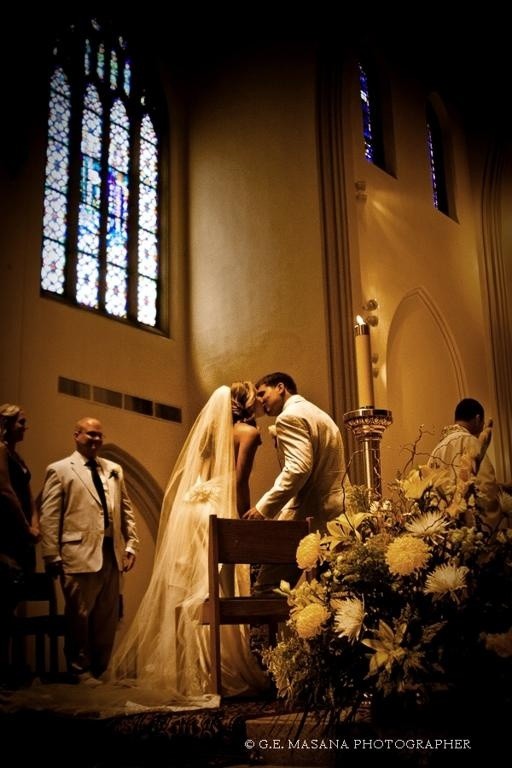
[
  {"x1": 242, "y1": 373, "x2": 352, "y2": 532},
  {"x1": 40, "y1": 417, "x2": 138, "y2": 689},
  {"x1": 0, "y1": 404, "x2": 42, "y2": 690},
  {"x1": 106, "y1": 380, "x2": 263, "y2": 697},
  {"x1": 427, "y1": 398, "x2": 506, "y2": 538}
]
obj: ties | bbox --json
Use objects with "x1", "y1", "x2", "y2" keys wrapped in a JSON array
[{"x1": 89, "y1": 459, "x2": 109, "y2": 530}]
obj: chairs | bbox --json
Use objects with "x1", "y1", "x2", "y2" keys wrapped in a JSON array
[
  {"x1": 19, "y1": 570, "x2": 71, "y2": 681},
  {"x1": 174, "y1": 512, "x2": 314, "y2": 695}
]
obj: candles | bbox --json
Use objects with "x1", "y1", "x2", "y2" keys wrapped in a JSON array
[{"x1": 352, "y1": 314, "x2": 375, "y2": 408}]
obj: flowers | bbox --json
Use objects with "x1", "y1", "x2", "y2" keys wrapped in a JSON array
[{"x1": 247, "y1": 420, "x2": 512, "y2": 723}]
